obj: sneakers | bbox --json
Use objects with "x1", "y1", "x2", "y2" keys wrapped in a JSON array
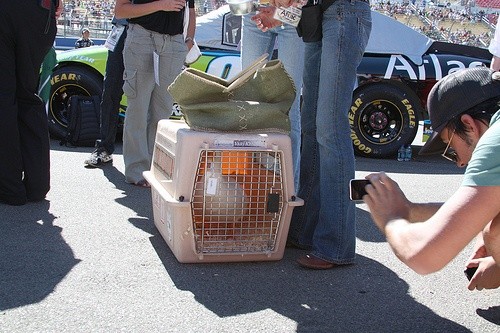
[{"x1": 84, "y1": 148, "x2": 114, "y2": 167}]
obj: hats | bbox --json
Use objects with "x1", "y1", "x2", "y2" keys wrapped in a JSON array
[{"x1": 421, "y1": 68, "x2": 500, "y2": 155}]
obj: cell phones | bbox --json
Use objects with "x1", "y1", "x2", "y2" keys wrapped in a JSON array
[{"x1": 349, "y1": 179, "x2": 371, "y2": 202}]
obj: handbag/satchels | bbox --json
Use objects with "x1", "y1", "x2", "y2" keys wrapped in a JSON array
[
  {"x1": 165, "y1": 53, "x2": 296, "y2": 135},
  {"x1": 295, "y1": 3, "x2": 323, "y2": 42}
]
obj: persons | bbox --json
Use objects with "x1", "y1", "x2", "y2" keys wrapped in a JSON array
[
  {"x1": 463, "y1": 243, "x2": 500, "y2": 291},
  {"x1": 250, "y1": 0, "x2": 371, "y2": 271},
  {"x1": 488, "y1": 12, "x2": 499, "y2": 73},
  {"x1": 0, "y1": 0, "x2": 59, "y2": 206},
  {"x1": 56, "y1": 0, "x2": 117, "y2": 22},
  {"x1": 241, "y1": 0, "x2": 307, "y2": 196},
  {"x1": 83, "y1": 18, "x2": 130, "y2": 167},
  {"x1": 364, "y1": 67, "x2": 500, "y2": 275},
  {"x1": 75, "y1": 29, "x2": 94, "y2": 48},
  {"x1": 113, "y1": 0, "x2": 197, "y2": 188},
  {"x1": 369, "y1": 0, "x2": 500, "y2": 49}
]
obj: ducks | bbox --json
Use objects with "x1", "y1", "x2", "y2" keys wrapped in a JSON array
[{"x1": 194, "y1": 152, "x2": 251, "y2": 225}]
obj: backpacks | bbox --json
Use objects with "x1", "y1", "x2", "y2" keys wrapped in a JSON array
[{"x1": 59, "y1": 94, "x2": 102, "y2": 147}]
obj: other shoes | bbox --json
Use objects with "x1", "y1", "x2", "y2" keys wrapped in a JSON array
[
  {"x1": 134, "y1": 178, "x2": 151, "y2": 187},
  {"x1": 295, "y1": 254, "x2": 336, "y2": 269}
]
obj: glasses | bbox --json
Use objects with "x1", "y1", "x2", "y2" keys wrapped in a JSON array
[{"x1": 441, "y1": 124, "x2": 459, "y2": 163}]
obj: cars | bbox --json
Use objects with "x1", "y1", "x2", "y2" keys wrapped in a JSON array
[{"x1": 47, "y1": 2, "x2": 492, "y2": 158}]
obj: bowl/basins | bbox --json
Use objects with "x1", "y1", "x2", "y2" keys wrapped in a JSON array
[{"x1": 227, "y1": 0, "x2": 258, "y2": 16}]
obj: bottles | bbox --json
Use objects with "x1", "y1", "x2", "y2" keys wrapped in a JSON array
[{"x1": 398, "y1": 143, "x2": 412, "y2": 162}]
